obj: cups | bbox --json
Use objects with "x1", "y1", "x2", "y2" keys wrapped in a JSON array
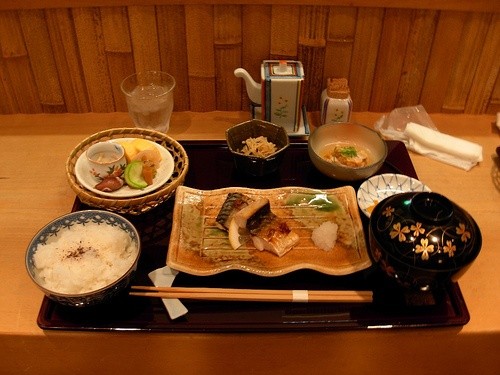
[
  {"x1": 225, "y1": 119, "x2": 291, "y2": 180},
  {"x1": 86, "y1": 142, "x2": 127, "y2": 183},
  {"x1": 120, "y1": 71, "x2": 176, "y2": 134}
]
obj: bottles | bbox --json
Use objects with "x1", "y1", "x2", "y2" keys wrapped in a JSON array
[{"x1": 320, "y1": 78, "x2": 353, "y2": 125}]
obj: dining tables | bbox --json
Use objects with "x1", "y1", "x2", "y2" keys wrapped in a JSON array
[{"x1": 1, "y1": 110, "x2": 500, "y2": 375}]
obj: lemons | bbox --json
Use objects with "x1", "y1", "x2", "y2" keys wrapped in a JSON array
[{"x1": 125, "y1": 160, "x2": 148, "y2": 188}]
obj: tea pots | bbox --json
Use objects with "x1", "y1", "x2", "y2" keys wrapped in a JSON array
[{"x1": 234, "y1": 59, "x2": 305, "y2": 132}]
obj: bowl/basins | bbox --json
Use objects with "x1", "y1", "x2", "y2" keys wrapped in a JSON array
[
  {"x1": 25, "y1": 210, "x2": 141, "y2": 307},
  {"x1": 308, "y1": 121, "x2": 388, "y2": 181},
  {"x1": 368, "y1": 191, "x2": 482, "y2": 290}
]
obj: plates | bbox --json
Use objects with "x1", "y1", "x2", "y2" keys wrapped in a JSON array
[
  {"x1": 165, "y1": 185, "x2": 373, "y2": 278},
  {"x1": 250, "y1": 103, "x2": 310, "y2": 137},
  {"x1": 75, "y1": 138, "x2": 174, "y2": 198},
  {"x1": 357, "y1": 173, "x2": 432, "y2": 219}
]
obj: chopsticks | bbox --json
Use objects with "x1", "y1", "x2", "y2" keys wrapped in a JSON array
[{"x1": 128, "y1": 285, "x2": 373, "y2": 304}]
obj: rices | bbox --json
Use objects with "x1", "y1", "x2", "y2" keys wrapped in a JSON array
[{"x1": 33, "y1": 223, "x2": 136, "y2": 293}]
